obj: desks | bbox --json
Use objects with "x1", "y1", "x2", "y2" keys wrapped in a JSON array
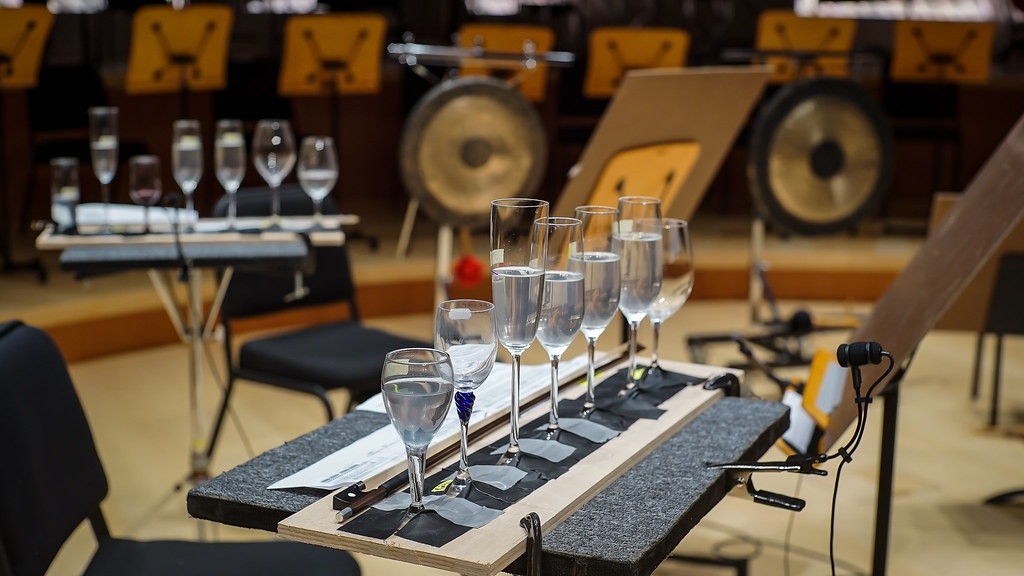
[
  {"x1": 187, "y1": 357, "x2": 789, "y2": 576},
  {"x1": 35, "y1": 220, "x2": 345, "y2": 462}
]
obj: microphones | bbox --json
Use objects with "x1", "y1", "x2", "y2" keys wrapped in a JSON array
[{"x1": 836, "y1": 341, "x2": 883, "y2": 368}]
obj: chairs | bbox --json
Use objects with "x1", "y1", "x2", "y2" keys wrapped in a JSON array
[
  {"x1": 205, "y1": 185, "x2": 434, "y2": 459},
  {"x1": 0, "y1": 321, "x2": 363, "y2": 576}
]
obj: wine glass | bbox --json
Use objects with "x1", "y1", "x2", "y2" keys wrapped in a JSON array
[
  {"x1": 381, "y1": 196, "x2": 695, "y2": 527},
  {"x1": 49, "y1": 105, "x2": 339, "y2": 235}
]
